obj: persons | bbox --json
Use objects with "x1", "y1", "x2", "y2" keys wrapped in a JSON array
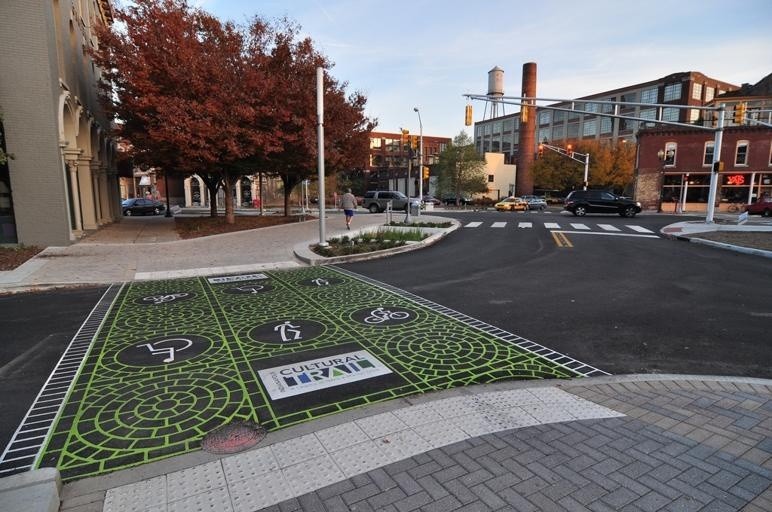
[
  {"x1": 155, "y1": 191, "x2": 161, "y2": 200},
  {"x1": 146, "y1": 191, "x2": 152, "y2": 200},
  {"x1": 338, "y1": 188, "x2": 358, "y2": 229}
]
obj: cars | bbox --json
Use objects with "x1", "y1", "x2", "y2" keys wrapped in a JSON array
[
  {"x1": 563, "y1": 190, "x2": 642, "y2": 218},
  {"x1": 417, "y1": 191, "x2": 473, "y2": 206},
  {"x1": 746, "y1": 197, "x2": 772, "y2": 217},
  {"x1": 121, "y1": 198, "x2": 165, "y2": 216},
  {"x1": 362, "y1": 190, "x2": 422, "y2": 213},
  {"x1": 494, "y1": 195, "x2": 547, "y2": 212}
]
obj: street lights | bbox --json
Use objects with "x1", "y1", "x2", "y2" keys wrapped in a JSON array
[
  {"x1": 657, "y1": 149, "x2": 675, "y2": 212},
  {"x1": 303, "y1": 178, "x2": 312, "y2": 210},
  {"x1": 414, "y1": 107, "x2": 423, "y2": 210},
  {"x1": 622, "y1": 140, "x2": 640, "y2": 201},
  {"x1": 159, "y1": 164, "x2": 173, "y2": 217}
]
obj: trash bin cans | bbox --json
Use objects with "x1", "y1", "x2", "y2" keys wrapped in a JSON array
[
  {"x1": 253, "y1": 199, "x2": 260, "y2": 208},
  {"x1": 411, "y1": 204, "x2": 421, "y2": 216}
]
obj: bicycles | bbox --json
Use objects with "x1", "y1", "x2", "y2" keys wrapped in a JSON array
[{"x1": 727, "y1": 201, "x2": 746, "y2": 213}]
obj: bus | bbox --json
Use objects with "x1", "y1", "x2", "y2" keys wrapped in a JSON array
[{"x1": 536, "y1": 189, "x2": 562, "y2": 206}]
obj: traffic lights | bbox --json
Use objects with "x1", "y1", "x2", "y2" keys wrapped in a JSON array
[
  {"x1": 538, "y1": 145, "x2": 544, "y2": 158},
  {"x1": 567, "y1": 144, "x2": 572, "y2": 156},
  {"x1": 732, "y1": 104, "x2": 745, "y2": 125},
  {"x1": 714, "y1": 161, "x2": 724, "y2": 173},
  {"x1": 401, "y1": 128, "x2": 419, "y2": 152},
  {"x1": 684, "y1": 176, "x2": 689, "y2": 181},
  {"x1": 423, "y1": 166, "x2": 430, "y2": 180}
]
